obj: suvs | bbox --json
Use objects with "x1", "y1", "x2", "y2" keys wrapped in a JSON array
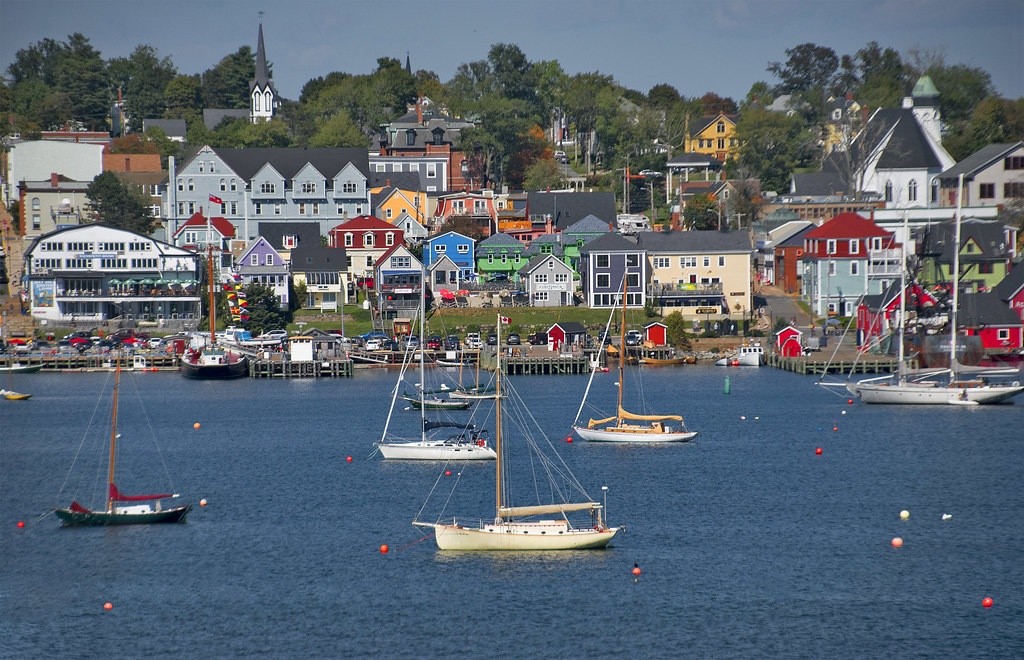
[
  {"x1": 527, "y1": 331, "x2": 548, "y2": 345},
  {"x1": 627, "y1": 329, "x2": 643, "y2": 345},
  {"x1": 257, "y1": 329, "x2": 288, "y2": 341}
]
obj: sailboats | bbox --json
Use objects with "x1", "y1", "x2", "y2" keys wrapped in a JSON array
[
  {"x1": 0, "y1": 309, "x2": 48, "y2": 374},
  {"x1": 371, "y1": 260, "x2": 503, "y2": 460},
  {"x1": 411, "y1": 312, "x2": 626, "y2": 551},
  {"x1": 180, "y1": 240, "x2": 249, "y2": 381},
  {"x1": 570, "y1": 264, "x2": 700, "y2": 443},
  {"x1": 814, "y1": 172, "x2": 1024, "y2": 408},
  {"x1": 49, "y1": 344, "x2": 194, "y2": 527}
]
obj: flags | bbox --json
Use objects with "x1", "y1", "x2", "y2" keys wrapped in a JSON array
[
  {"x1": 500, "y1": 315, "x2": 512, "y2": 324},
  {"x1": 208, "y1": 194, "x2": 223, "y2": 204},
  {"x1": 224, "y1": 271, "x2": 250, "y2": 323}
]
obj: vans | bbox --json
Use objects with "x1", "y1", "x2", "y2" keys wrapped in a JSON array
[
  {"x1": 464, "y1": 333, "x2": 479, "y2": 345},
  {"x1": 401, "y1": 337, "x2": 420, "y2": 351}
]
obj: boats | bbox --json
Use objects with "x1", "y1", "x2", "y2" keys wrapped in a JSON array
[
  {"x1": 0, "y1": 390, "x2": 34, "y2": 400},
  {"x1": 641, "y1": 344, "x2": 687, "y2": 365},
  {"x1": 714, "y1": 346, "x2": 766, "y2": 367}
]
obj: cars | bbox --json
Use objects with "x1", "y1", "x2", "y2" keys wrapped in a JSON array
[
  {"x1": 380, "y1": 340, "x2": 399, "y2": 351},
  {"x1": 624, "y1": 334, "x2": 640, "y2": 347},
  {"x1": 468, "y1": 338, "x2": 483, "y2": 350},
  {"x1": 638, "y1": 170, "x2": 663, "y2": 179},
  {"x1": 444, "y1": 335, "x2": 460, "y2": 350},
  {"x1": 366, "y1": 339, "x2": 382, "y2": 351},
  {"x1": 358, "y1": 334, "x2": 369, "y2": 342},
  {"x1": 506, "y1": 333, "x2": 520, "y2": 345},
  {"x1": 554, "y1": 150, "x2": 570, "y2": 165},
  {"x1": 56, "y1": 330, "x2": 164, "y2": 349},
  {"x1": 488, "y1": 333, "x2": 497, "y2": 345},
  {"x1": 426, "y1": 336, "x2": 442, "y2": 349}
]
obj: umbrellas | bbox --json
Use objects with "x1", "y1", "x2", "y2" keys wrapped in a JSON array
[
  {"x1": 826, "y1": 318, "x2": 842, "y2": 328},
  {"x1": 464, "y1": 272, "x2": 478, "y2": 283},
  {"x1": 510, "y1": 291, "x2": 528, "y2": 295},
  {"x1": 490, "y1": 272, "x2": 507, "y2": 278},
  {"x1": 107, "y1": 277, "x2": 200, "y2": 285},
  {"x1": 439, "y1": 289, "x2": 471, "y2": 300}
]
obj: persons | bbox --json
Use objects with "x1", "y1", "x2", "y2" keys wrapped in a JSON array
[
  {"x1": 809, "y1": 323, "x2": 814, "y2": 336},
  {"x1": 822, "y1": 324, "x2": 826, "y2": 335}
]
